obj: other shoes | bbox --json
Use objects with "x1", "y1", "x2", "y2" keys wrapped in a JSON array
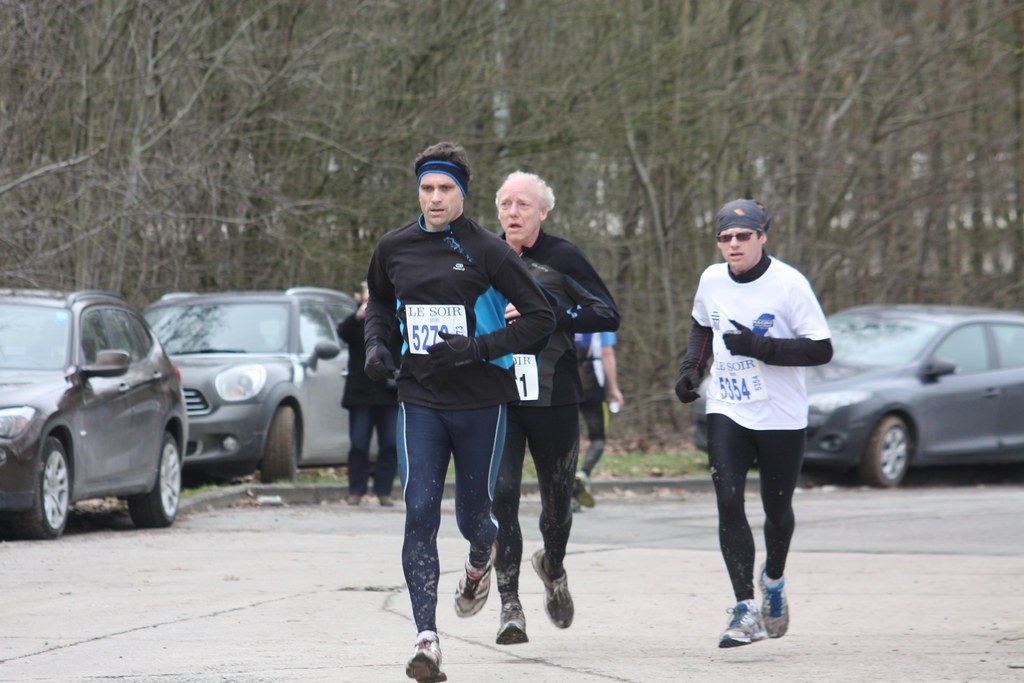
[
  {"x1": 570, "y1": 498, "x2": 581, "y2": 512},
  {"x1": 347, "y1": 494, "x2": 361, "y2": 505},
  {"x1": 377, "y1": 494, "x2": 395, "y2": 506},
  {"x1": 573, "y1": 468, "x2": 594, "y2": 508}
]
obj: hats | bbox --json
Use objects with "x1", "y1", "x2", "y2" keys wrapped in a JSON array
[{"x1": 716, "y1": 198, "x2": 770, "y2": 235}]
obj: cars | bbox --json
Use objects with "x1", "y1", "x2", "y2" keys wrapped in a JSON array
[
  {"x1": 691, "y1": 303, "x2": 1024, "y2": 491},
  {"x1": 0, "y1": 285, "x2": 189, "y2": 543},
  {"x1": 139, "y1": 285, "x2": 380, "y2": 486}
]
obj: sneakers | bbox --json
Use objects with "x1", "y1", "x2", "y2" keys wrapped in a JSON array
[
  {"x1": 719, "y1": 603, "x2": 767, "y2": 648},
  {"x1": 758, "y1": 562, "x2": 789, "y2": 639},
  {"x1": 454, "y1": 541, "x2": 497, "y2": 617},
  {"x1": 530, "y1": 548, "x2": 574, "y2": 629},
  {"x1": 496, "y1": 599, "x2": 528, "y2": 645},
  {"x1": 406, "y1": 630, "x2": 447, "y2": 683}
]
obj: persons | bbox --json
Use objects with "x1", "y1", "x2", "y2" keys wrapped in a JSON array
[
  {"x1": 364, "y1": 141, "x2": 556, "y2": 683},
  {"x1": 570, "y1": 331, "x2": 624, "y2": 512},
  {"x1": 492, "y1": 171, "x2": 620, "y2": 644},
  {"x1": 337, "y1": 284, "x2": 399, "y2": 507},
  {"x1": 675, "y1": 199, "x2": 835, "y2": 648}
]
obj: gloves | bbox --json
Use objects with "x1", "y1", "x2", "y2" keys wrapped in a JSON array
[
  {"x1": 427, "y1": 331, "x2": 488, "y2": 374},
  {"x1": 723, "y1": 320, "x2": 754, "y2": 357},
  {"x1": 675, "y1": 369, "x2": 700, "y2": 404},
  {"x1": 364, "y1": 336, "x2": 396, "y2": 382}
]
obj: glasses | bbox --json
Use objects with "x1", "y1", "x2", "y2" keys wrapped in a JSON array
[{"x1": 716, "y1": 230, "x2": 759, "y2": 243}]
